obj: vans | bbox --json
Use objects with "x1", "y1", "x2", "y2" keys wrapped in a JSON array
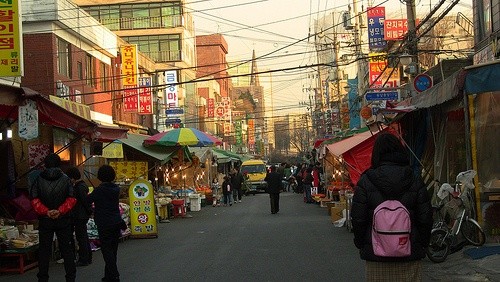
[{"x1": 239, "y1": 160, "x2": 268, "y2": 196}]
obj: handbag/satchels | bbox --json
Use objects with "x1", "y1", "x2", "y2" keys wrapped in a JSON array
[{"x1": 118, "y1": 219, "x2": 127, "y2": 232}]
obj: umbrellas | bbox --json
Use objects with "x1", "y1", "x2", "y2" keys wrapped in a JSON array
[
  {"x1": 215, "y1": 150, "x2": 240, "y2": 174},
  {"x1": 142, "y1": 127, "x2": 222, "y2": 218}
]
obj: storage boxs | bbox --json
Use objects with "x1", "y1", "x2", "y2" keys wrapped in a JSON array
[
  {"x1": 331, "y1": 207, "x2": 344, "y2": 223},
  {"x1": 190, "y1": 194, "x2": 202, "y2": 211}
]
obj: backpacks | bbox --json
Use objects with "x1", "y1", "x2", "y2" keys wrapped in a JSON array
[{"x1": 361, "y1": 166, "x2": 421, "y2": 257}]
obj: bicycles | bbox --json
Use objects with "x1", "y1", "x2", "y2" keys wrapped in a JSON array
[{"x1": 426, "y1": 170, "x2": 487, "y2": 263}]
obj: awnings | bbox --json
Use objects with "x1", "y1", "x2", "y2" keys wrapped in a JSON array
[{"x1": 37, "y1": 94, "x2": 129, "y2": 142}]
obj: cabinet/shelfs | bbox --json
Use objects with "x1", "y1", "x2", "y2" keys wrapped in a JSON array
[{"x1": 0, "y1": 237, "x2": 39, "y2": 274}]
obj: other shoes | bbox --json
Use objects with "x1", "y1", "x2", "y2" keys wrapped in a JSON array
[
  {"x1": 102, "y1": 276, "x2": 120, "y2": 282},
  {"x1": 89, "y1": 260, "x2": 93, "y2": 263},
  {"x1": 75, "y1": 261, "x2": 88, "y2": 266}
]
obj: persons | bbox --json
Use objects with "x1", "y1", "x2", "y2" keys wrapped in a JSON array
[
  {"x1": 30, "y1": 152, "x2": 77, "y2": 282},
  {"x1": 277, "y1": 161, "x2": 321, "y2": 205},
  {"x1": 264, "y1": 165, "x2": 282, "y2": 215},
  {"x1": 211, "y1": 167, "x2": 244, "y2": 207},
  {"x1": 351, "y1": 131, "x2": 433, "y2": 282},
  {"x1": 66, "y1": 166, "x2": 93, "y2": 267},
  {"x1": 87, "y1": 164, "x2": 127, "y2": 282}
]
